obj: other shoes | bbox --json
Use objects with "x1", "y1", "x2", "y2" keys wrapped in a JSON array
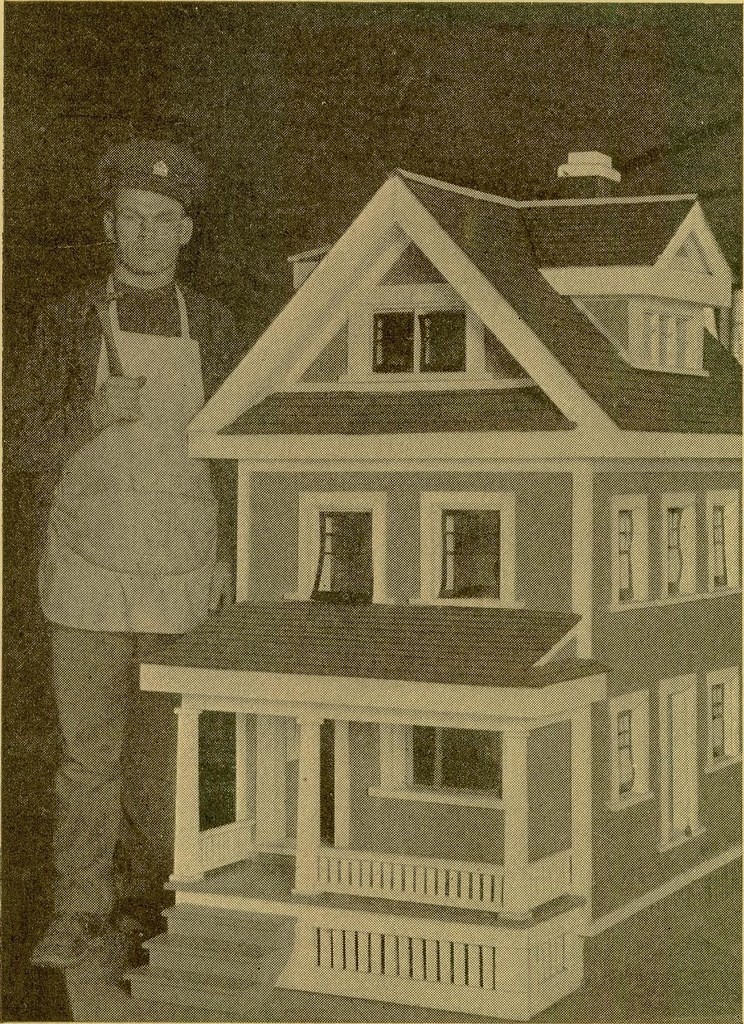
[
  {"x1": 31, "y1": 914, "x2": 109, "y2": 968},
  {"x1": 118, "y1": 894, "x2": 174, "y2": 935}
]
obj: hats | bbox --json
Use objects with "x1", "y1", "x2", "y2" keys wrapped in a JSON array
[{"x1": 101, "y1": 141, "x2": 205, "y2": 214}]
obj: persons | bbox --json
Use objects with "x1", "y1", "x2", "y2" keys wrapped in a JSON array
[{"x1": 8, "y1": 135, "x2": 241, "y2": 965}]
